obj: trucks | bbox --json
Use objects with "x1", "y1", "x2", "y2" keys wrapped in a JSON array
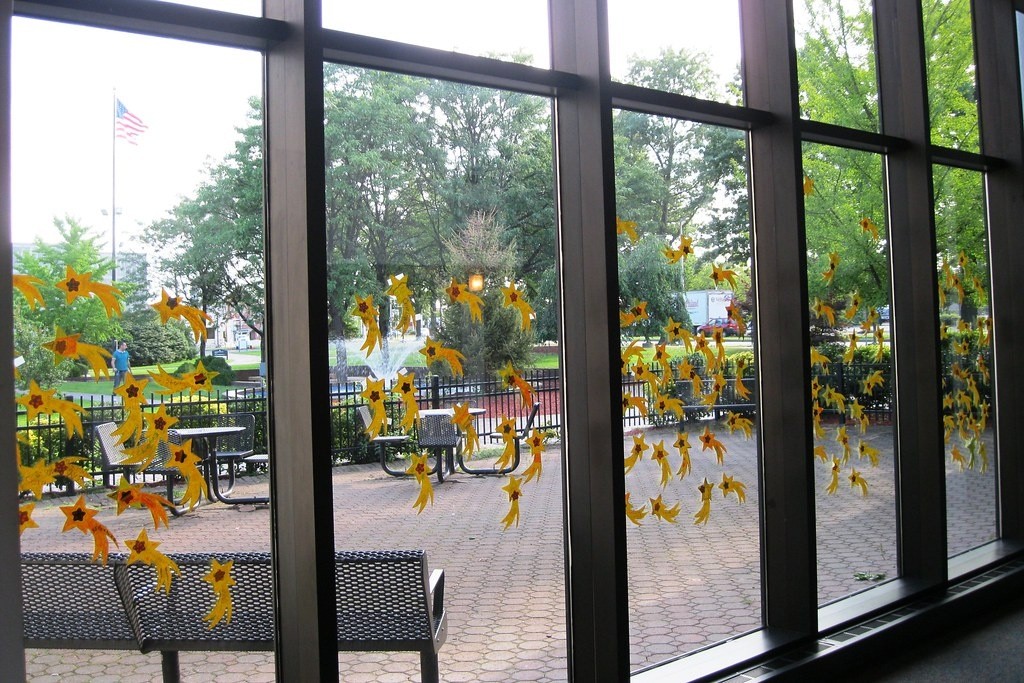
[{"x1": 672, "y1": 290, "x2": 734, "y2": 335}]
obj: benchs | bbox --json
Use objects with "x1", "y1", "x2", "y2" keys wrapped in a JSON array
[
  {"x1": 674, "y1": 379, "x2": 757, "y2": 431},
  {"x1": 18, "y1": 548, "x2": 448, "y2": 683}
]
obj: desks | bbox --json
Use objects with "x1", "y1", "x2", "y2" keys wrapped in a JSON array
[
  {"x1": 415, "y1": 409, "x2": 486, "y2": 482},
  {"x1": 141, "y1": 426, "x2": 246, "y2": 512}
]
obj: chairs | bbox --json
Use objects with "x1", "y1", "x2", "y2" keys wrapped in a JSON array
[
  {"x1": 356, "y1": 406, "x2": 411, "y2": 477},
  {"x1": 136, "y1": 429, "x2": 182, "y2": 517},
  {"x1": 443, "y1": 400, "x2": 478, "y2": 467},
  {"x1": 245, "y1": 452, "x2": 270, "y2": 505},
  {"x1": 208, "y1": 412, "x2": 255, "y2": 504},
  {"x1": 414, "y1": 414, "x2": 456, "y2": 484},
  {"x1": 490, "y1": 401, "x2": 542, "y2": 474},
  {"x1": 96, "y1": 422, "x2": 162, "y2": 485}
]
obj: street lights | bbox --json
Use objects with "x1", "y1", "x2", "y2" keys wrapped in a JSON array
[{"x1": 679, "y1": 215, "x2": 690, "y2": 309}]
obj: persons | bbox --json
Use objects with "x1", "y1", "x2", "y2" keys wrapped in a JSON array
[{"x1": 111, "y1": 340, "x2": 133, "y2": 394}]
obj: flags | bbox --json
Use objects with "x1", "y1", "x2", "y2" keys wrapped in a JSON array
[{"x1": 115, "y1": 98, "x2": 149, "y2": 146}]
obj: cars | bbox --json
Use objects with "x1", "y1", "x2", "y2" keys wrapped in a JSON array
[
  {"x1": 878, "y1": 307, "x2": 889, "y2": 325},
  {"x1": 697, "y1": 318, "x2": 747, "y2": 337}
]
